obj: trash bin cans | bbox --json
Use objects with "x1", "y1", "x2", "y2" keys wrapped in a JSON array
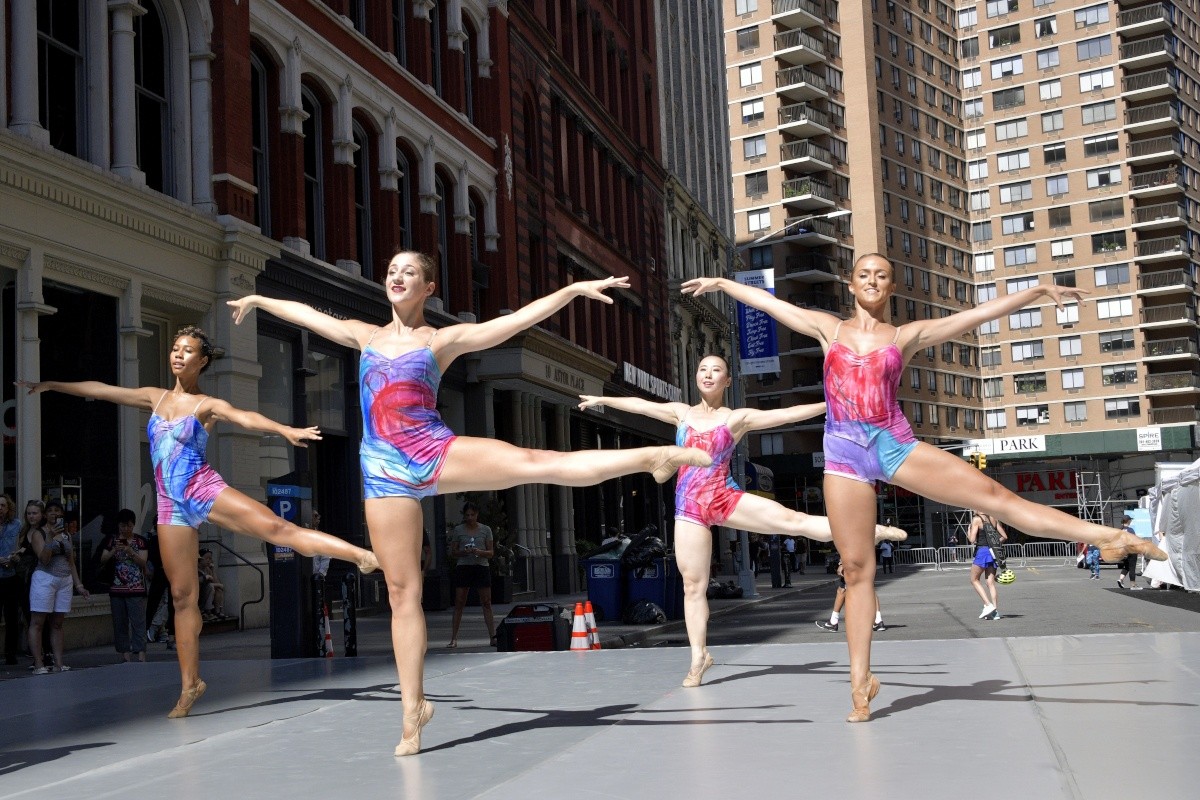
[
  {"x1": 578, "y1": 540, "x2": 627, "y2": 623},
  {"x1": 627, "y1": 550, "x2": 684, "y2": 621}
]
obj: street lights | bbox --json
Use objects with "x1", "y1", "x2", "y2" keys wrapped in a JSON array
[{"x1": 727, "y1": 209, "x2": 855, "y2": 600}]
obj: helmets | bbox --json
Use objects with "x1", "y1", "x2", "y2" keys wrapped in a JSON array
[{"x1": 995, "y1": 569, "x2": 1016, "y2": 585}]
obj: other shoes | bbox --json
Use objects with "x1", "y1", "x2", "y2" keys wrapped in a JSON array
[
  {"x1": 1096, "y1": 576, "x2": 1100, "y2": 580},
  {"x1": 978, "y1": 603, "x2": 1000, "y2": 620},
  {"x1": 1090, "y1": 575, "x2": 1095, "y2": 579},
  {"x1": 1117, "y1": 579, "x2": 1125, "y2": 588},
  {"x1": 1130, "y1": 584, "x2": 1143, "y2": 590}
]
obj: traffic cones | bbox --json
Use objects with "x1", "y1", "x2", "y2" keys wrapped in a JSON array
[
  {"x1": 322, "y1": 601, "x2": 336, "y2": 657},
  {"x1": 569, "y1": 600, "x2": 602, "y2": 651}
]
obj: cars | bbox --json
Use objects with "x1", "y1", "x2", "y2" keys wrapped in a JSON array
[{"x1": 1076, "y1": 541, "x2": 1129, "y2": 569}]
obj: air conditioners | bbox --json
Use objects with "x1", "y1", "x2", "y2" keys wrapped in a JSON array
[
  {"x1": 1174, "y1": 341, "x2": 1186, "y2": 354},
  {"x1": 838, "y1": 231, "x2": 848, "y2": 240},
  {"x1": 841, "y1": 268, "x2": 850, "y2": 278},
  {"x1": 832, "y1": 123, "x2": 843, "y2": 131},
  {"x1": 825, "y1": 15, "x2": 836, "y2": 25},
  {"x1": 836, "y1": 159, "x2": 844, "y2": 169},
  {"x1": 831, "y1": 86, "x2": 839, "y2": 97},
  {"x1": 837, "y1": 194, "x2": 849, "y2": 208},
  {"x1": 843, "y1": 304, "x2": 853, "y2": 315},
  {"x1": 829, "y1": 53, "x2": 835, "y2": 65}
]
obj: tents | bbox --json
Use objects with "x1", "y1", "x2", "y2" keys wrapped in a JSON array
[{"x1": 1137, "y1": 456, "x2": 1200, "y2": 594}]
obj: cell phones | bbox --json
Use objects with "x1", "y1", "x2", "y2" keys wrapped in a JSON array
[{"x1": 56, "y1": 516, "x2": 64, "y2": 529}]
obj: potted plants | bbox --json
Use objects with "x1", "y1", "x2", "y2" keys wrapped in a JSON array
[
  {"x1": 1095, "y1": 240, "x2": 1127, "y2": 255},
  {"x1": 1170, "y1": 234, "x2": 1184, "y2": 252},
  {"x1": 1000, "y1": 37, "x2": 1011, "y2": 47},
  {"x1": 1151, "y1": 345, "x2": 1164, "y2": 356}
]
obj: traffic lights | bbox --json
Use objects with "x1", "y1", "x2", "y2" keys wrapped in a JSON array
[
  {"x1": 979, "y1": 453, "x2": 988, "y2": 470},
  {"x1": 969, "y1": 454, "x2": 976, "y2": 468}
]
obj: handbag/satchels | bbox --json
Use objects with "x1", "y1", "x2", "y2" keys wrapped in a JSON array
[{"x1": 890, "y1": 545, "x2": 895, "y2": 552}]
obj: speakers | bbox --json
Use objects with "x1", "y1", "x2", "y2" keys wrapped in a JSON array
[{"x1": 495, "y1": 603, "x2": 569, "y2": 652}]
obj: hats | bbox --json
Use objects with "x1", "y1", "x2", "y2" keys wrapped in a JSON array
[{"x1": 1122, "y1": 515, "x2": 1133, "y2": 520}]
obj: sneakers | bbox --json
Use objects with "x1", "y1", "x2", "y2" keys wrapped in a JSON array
[
  {"x1": 814, "y1": 619, "x2": 838, "y2": 632},
  {"x1": 872, "y1": 620, "x2": 886, "y2": 631}
]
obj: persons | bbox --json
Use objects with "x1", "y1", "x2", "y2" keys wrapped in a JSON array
[
  {"x1": 948, "y1": 534, "x2": 958, "y2": 563},
  {"x1": 225, "y1": 249, "x2": 715, "y2": 756},
  {"x1": 1116, "y1": 515, "x2": 1143, "y2": 591},
  {"x1": 12, "y1": 325, "x2": 383, "y2": 719},
  {"x1": 1080, "y1": 543, "x2": 1102, "y2": 581},
  {"x1": 446, "y1": 502, "x2": 498, "y2": 649},
  {"x1": 601, "y1": 526, "x2": 619, "y2": 546},
  {"x1": 815, "y1": 556, "x2": 885, "y2": 633},
  {"x1": 748, "y1": 532, "x2": 808, "y2": 579},
  {"x1": 421, "y1": 529, "x2": 434, "y2": 607},
  {"x1": 577, "y1": 355, "x2": 908, "y2": 688},
  {"x1": 967, "y1": 510, "x2": 1007, "y2": 620},
  {"x1": 879, "y1": 539, "x2": 894, "y2": 575},
  {"x1": 680, "y1": 253, "x2": 1168, "y2": 722},
  {"x1": 0, "y1": 493, "x2": 331, "y2": 683}
]
